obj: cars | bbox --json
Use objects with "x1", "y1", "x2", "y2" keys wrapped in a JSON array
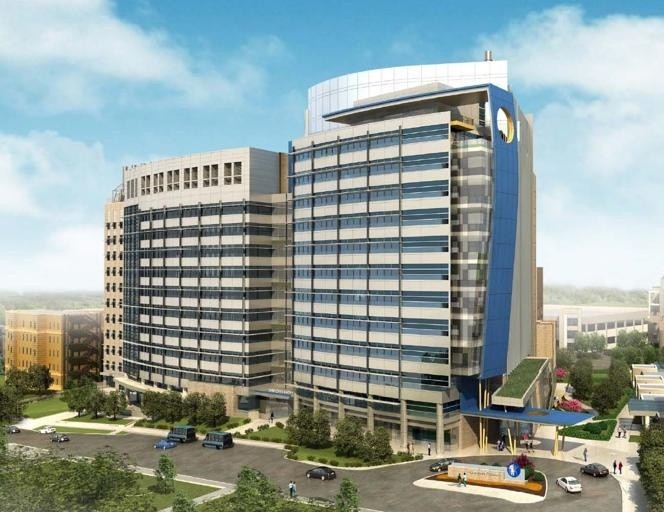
[
  {"x1": 429, "y1": 458, "x2": 458, "y2": 472},
  {"x1": 39, "y1": 426, "x2": 69, "y2": 442},
  {"x1": 7, "y1": 426, "x2": 20, "y2": 434},
  {"x1": 153, "y1": 439, "x2": 176, "y2": 449},
  {"x1": 581, "y1": 463, "x2": 609, "y2": 477},
  {"x1": 306, "y1": 467, "x2": 336, "y2": 480},
  {"x1": 556, "y1": 477, "x2": 582, "y2": 493}
]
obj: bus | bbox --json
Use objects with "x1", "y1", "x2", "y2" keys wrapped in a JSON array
[
  {"x1": 167, "y1": 424, "x2": 195, "y2": 442},
  {"x1": 200, "y1": 432, "x2": 234, "y2": 449}
]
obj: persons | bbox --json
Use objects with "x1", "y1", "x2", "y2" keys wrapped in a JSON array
[
  {"x1": 407, "y1": 441, "x2": 410, "y2": 455},
  {"x1": 457, "y1": 473, "x2": 462, "y2": 486},
  {"x1": 288, "y1": 479, "x2": 292, "y2": 497},
  {"x1": 411, "y1": 442, "x2": 415, "y2": 455},
  {"x1": 496, "y1": 430, "x2": 506, "y2": 452},
  {"x1": 617, "y1": 425, "x2": 622, "y2": 438},
  {"x1": 613, "y1": 459, "x2": 617, "y2": 474},
  {"x1": 528, "y1": 433, "x2": 534, "y2": 454},
  {"x1": 427, "y1": 442, "x2": 431, "y2": 456},
  {"x1": 292, "y1": 481, "x2": 297, "y2": 498},
  {"x1": 583, "y1": 447, "x2": 588, "y2": 461},
  {"x1": 623, "y1": 425, "x2": 626, "y2": 437},
  {"x1": 463, "y1": 473, "x2": 467, "y2": 488},
  {"x1": 523, "y1": 433, "x2": 528, "y2": 450},
  {"x1": 618, "y1": 461, "x2": 624, "y2": 475},
  {"x1": 270, "y1": 410, "x2": 274, "y2": 423}
]
